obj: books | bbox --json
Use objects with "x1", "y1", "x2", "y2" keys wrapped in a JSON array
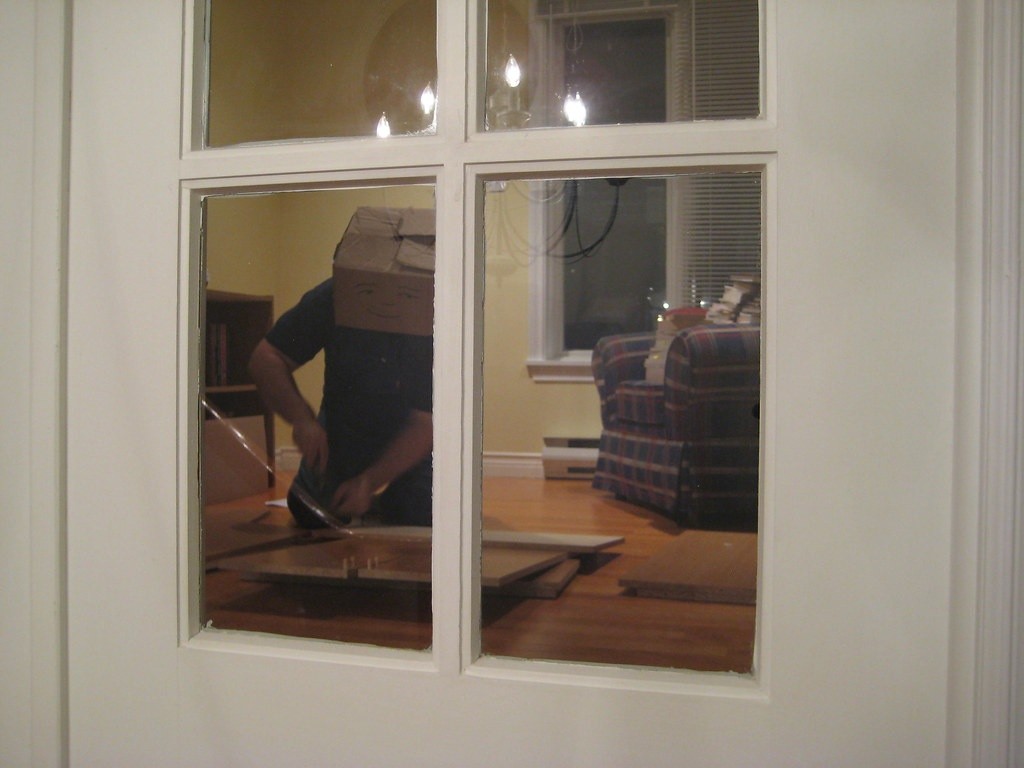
[{"x1": 204, "y1": 320, "x2": 228, "y2": 385}]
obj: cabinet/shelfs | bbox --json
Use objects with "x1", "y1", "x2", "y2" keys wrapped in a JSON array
[{"x1": 201, "y1": 289, "x2": 276, "y2": 508}]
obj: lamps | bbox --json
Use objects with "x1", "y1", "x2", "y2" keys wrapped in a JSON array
[{"x1": 367, "y1": 0, "x2": 589, "y2": 206}]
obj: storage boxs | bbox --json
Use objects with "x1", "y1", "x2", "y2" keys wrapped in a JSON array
[{"x1": 333, "y1": 207, "x2": 435, "y2": 337}]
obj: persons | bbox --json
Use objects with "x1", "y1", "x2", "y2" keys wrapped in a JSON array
[{"x1": 249, "y1": 207, "x2": 433, "y2": 529}]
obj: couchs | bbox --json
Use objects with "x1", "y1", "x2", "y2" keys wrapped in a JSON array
[{"x1": 590, "y1": 324, "x2": 760, "y2": 528}]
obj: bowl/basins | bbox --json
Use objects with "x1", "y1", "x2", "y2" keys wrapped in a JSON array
[{"x1": 288, "y1": 461, "x2": 354, "y2": 528}]
